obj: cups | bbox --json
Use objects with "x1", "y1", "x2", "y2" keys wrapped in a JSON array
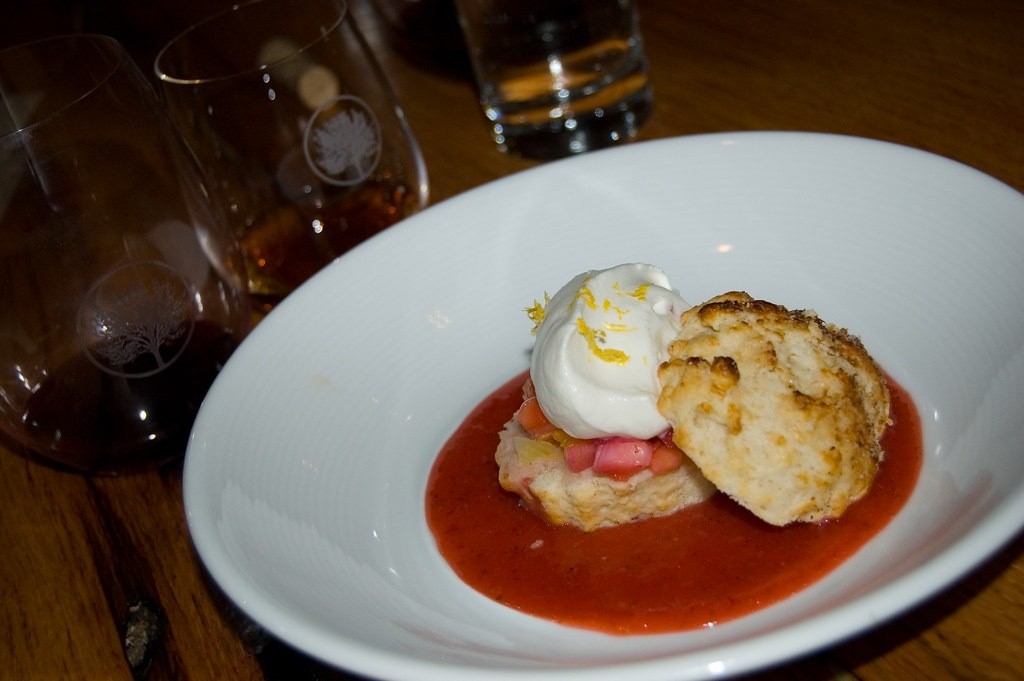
[
  {"x1": 0, "y1": 30, "x2": 246, "y2": 476},
  {"x1": 152, "y1": 0, "x2": 434, "y2": 318},
  {"x1": 453, "y1": 0, "x2": 660, "y2": 164}
]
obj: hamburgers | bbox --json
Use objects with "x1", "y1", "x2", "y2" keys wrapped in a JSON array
[{"x1": 494, "y1": 262, "x2": 894, "y2": 527}]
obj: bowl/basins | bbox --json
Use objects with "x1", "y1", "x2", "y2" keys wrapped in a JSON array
[{"x1": 181, "y1": 128, "x2": 1024, "y2": 680}]
